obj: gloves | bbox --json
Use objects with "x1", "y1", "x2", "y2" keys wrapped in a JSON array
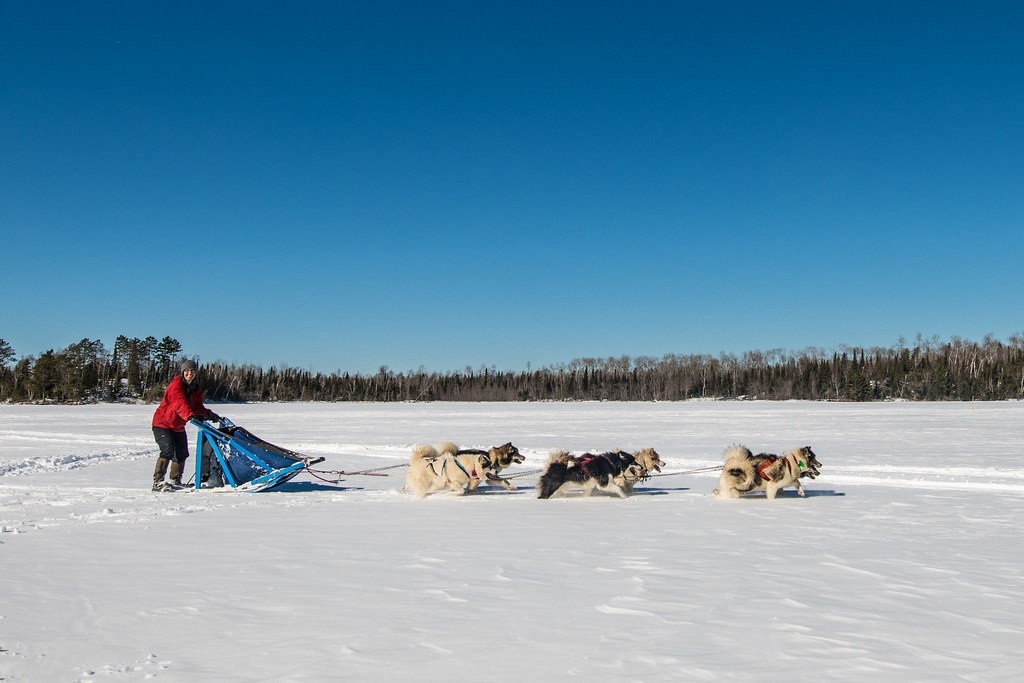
[
  {"x1": 208, "y1": 412, "x2": 219, "y2": 420},
  {"x1": 190, "y1": 415, "x2": 203, "y2": 422}
]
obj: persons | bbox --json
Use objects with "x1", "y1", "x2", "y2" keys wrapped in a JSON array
[{"x1": 152, "y1": 361, "x2": 221, "y2": 493}]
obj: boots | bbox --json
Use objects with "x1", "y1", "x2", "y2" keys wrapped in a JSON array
[
  {"x1": 170, "y1": 462, "x2": 195, "y2": 489},
  {"x1": 152, "y1": 458, "x2": 175, "y2": 493}
]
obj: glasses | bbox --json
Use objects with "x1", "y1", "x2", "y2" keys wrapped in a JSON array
[{"x1": 185, "y1": 369, "x2": 196, "y2": 373}]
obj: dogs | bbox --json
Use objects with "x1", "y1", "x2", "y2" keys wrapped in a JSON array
[
  {"x1": 714, "y1": 442, "x2": 823, "y2": 499},
  {"x1": 538, "y1": 447, "x2": 666, "y2": 500},
  {"x1": 402, "y1": 441, "x2": 525, "y2": 498}
]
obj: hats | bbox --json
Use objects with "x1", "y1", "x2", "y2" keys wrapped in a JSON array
[{"x1": 181, "y1": 360, "x2": 196, "y2": 375}]
